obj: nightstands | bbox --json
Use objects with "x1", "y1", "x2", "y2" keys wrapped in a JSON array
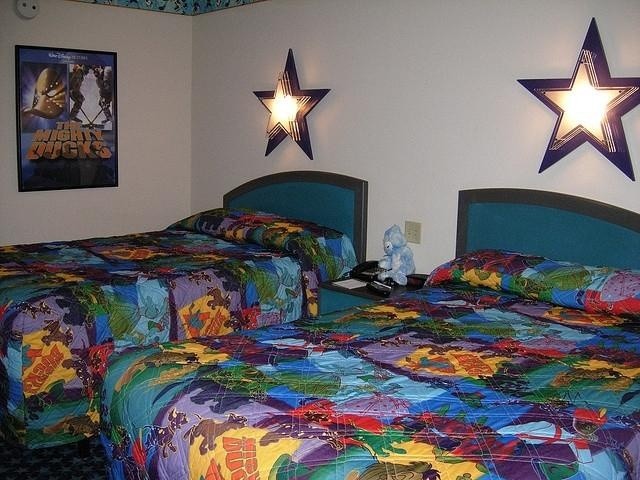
[{"x1": 318, "y1": 277, "x2": 427, "y2": 315}]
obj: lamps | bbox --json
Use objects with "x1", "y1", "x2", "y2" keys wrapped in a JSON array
[
  {"x1": 252, "y1": 48, "x2": 331, "y2": 160},
  {"x1": 515, "y1": 17, "x2": 640, "y2": 182}
]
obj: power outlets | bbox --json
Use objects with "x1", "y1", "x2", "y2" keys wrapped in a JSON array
[{"x1": 405, "y1": 220, "x2": 421, "y2": 244}]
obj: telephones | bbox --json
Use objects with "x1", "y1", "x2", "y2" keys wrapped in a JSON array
[{"x1": 351, "y1": 261, "x2": 384, "y2": 282}]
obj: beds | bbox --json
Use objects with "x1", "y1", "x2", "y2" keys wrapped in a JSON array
[
  {"x1": 72, "y1": 190, "x2": 640, "y2": 480},
  {"x1": 0, "y1": 167, "x2": 368, "y2": 449}
]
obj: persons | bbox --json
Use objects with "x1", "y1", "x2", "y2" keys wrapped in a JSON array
[
  {"x1": 70, "y1": 64, "x2": 90, "y2": 123},
  {"x1": 90, "y1": 63, "x2": 113, "y2": 123}
]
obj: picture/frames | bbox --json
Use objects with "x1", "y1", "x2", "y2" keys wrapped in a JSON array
[{"x1": 15, "y1": 45, "x2": 119, "y2": 192}]
